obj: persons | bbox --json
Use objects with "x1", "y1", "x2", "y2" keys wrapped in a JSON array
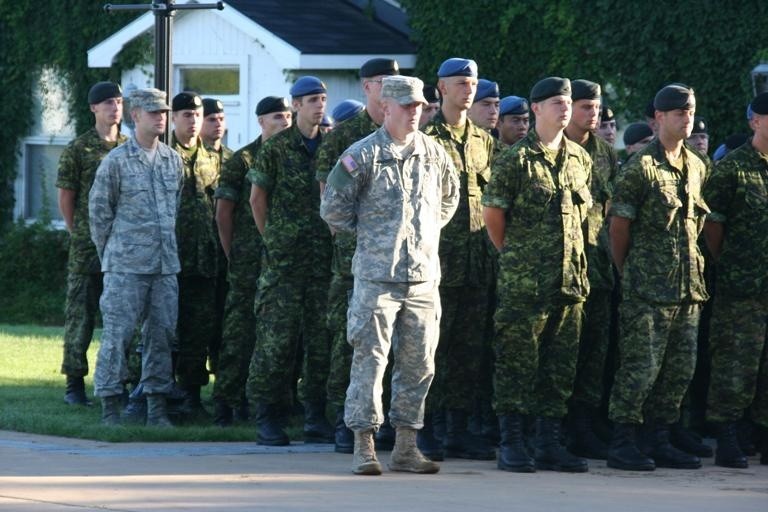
[{"x1": 55, "y1": 53, "x2": 768, "y2": 476}]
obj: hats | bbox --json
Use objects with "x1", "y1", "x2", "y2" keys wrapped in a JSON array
[
  {"x1": 422, "y1": 86, "x2": 442, "y2": 104},
  {"x1": 438, "y1": 58, "x2": 479, "y2": 77},
  {"x1": 500, "y1": 96, "x2": 530, "y2": 115},
  {"x1": 530, "y1": 77, "x2": 572, "y2": 103},
  {"x1": 289, "y1": 76, "x2": 327, "y2": 97},
  {"x1": 473, "y1": 79, "x2": 500, "y2": 103},
  {"x1": 257, "y1": 95, "x2": 289, "y2": 116},
  {"x1": 751, "y1": 92, "x2": 768, "y2": 114},
  {"x1": 624, "y1": 85, "x2": 710, "y2": 143},
  {"x1": 381, "y1": 75, "x2": 429, "y2": 106},
  {"x1": 333, "y1": 99, "x2": 367, "y2": 121},
  {"x1": 601, "y1": 106, "x2": 614, "y2": 120},
  {"x1": 359, "y1": 59, "x2": 399, "y2": 78},
  {"x1": 90, "y1": 80, "x2": 224, "y2": 112},
  {"x1": 571, "y1": 79, "x2": 601, "y2": 101}
]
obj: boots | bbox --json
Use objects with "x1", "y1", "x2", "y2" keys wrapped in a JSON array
[
  {"x1": 334, "y1": 408, "x2": 766, "y2": 474},
  {"x1": 302, "y1": 398, "x2": 335, "y2": 443},
  {"x1": 257, "y1": 404, "x2": 290, "y2": 444},
  {"x1": 65, "y1": 382, "x2": 250, "y2": 428}
]
